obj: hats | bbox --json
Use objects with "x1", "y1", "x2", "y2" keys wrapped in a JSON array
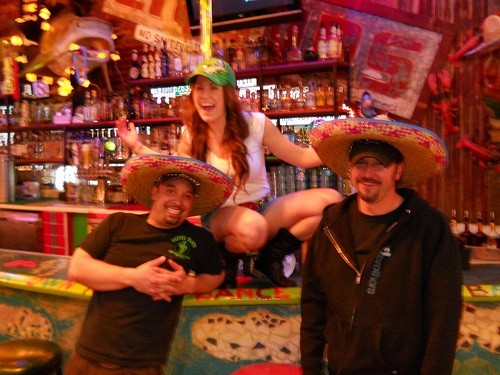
[
  {"x1": 349, "y1": 139, "x2": 403, "y2": 168},
  {"x1": 187, "y1": 58, "x2": 236, "y2": 89},
  {"x1": 121, "y1": 153, "x2": 234, "y2": 216},
  {"x1": 159, "y1": 172, "x2": 200, "y2": 193},
  {"x1": 310, "y1": 115, "x2": 449, "y2": 189}
]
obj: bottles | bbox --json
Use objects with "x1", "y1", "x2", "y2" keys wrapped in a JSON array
[
  {"x1": 0, "y1": 39, "x2": 201, "y2": 206},
  {"x1": 447, "y1": 209, "x2": 500, "y2": 250},
  {"x1": 278, "y1": 124, "x2": 312, "y2": 147},
  {"x1": 235, "y1": 77, "x2": 336, "y2": 112},
  {"x1": 212, "y1": 23, "x2": 343, "y2": 72}
]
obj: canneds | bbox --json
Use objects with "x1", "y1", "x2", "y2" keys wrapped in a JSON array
[{"x1": 267, "y1": 165, "x2": 335, "y2": 198}]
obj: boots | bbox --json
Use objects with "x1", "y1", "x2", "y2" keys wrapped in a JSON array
[
  {"x1": 219, "y1": 240, "x2": 248, "y2": 288},
  {"x1": 251, "y1": 227, "x2": 303, "y2": 287}
]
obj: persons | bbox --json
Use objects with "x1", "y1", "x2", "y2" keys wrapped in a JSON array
[
  {"x1": 62, "y1": 153, "x2": 234, "y2": 375},
  {"x1": 115, "y1": 58, "x2": 344, "y2": 290},
  {"x1": 299, "y1": 113, "x2": 464, "y2": 375}
]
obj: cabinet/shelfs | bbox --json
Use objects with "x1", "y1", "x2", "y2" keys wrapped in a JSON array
[{"x1": 0, "y1": 10, "x2": 351, "y2": 205}]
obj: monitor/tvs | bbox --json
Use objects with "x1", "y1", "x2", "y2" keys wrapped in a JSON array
[{"x1": 186, "y1": 0, "x2": 303, "y2": 31}]
